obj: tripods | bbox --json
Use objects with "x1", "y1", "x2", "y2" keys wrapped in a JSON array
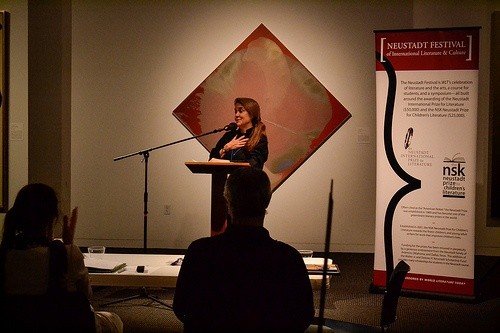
[{"x1": 99, "y1": 126, "x2": 228, "y2": 309}]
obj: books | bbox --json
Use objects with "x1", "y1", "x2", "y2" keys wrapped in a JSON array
[
  {"x1": 302, "y1": 257, "x2": 341, "y2": 275},
  {"x1": 81, "y1": 255, "x2": 126, "y2": 274}
]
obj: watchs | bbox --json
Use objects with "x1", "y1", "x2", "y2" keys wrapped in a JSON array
[{"x1": 222, "y1": 147, "x2": 227, "y2": 153}]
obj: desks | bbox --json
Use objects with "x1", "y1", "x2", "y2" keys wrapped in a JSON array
[{"x1": 82, "y1": 253, "x2": 330, "y2": 290}]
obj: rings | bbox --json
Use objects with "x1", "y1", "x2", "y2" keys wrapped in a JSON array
[{"x1": 239, "y1": 139, "x2": 242, "y2": 142}]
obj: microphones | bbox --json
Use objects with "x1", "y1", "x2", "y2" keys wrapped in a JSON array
[{"x1": 225, "y1": 122, "x2": 237, "y2": 131}]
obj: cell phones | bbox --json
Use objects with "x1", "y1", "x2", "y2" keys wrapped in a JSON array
[{"x1": 137, "y1": 266, "x2": 144, "y2": 272}]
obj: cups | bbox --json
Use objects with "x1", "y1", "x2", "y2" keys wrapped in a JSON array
[
  {"x1": 88, "y1": 246, "x2": 105, "y2": 257},
  {"x1": 298, "y1": 250, "x2": 313, "y2": 258}
]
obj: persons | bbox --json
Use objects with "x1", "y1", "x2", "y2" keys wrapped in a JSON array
[
  {"x1": 171, "y1": 166, "x2": 315, "y2": 333},
  {"x1": 208, "y1": 96, "x2": 269, "y2": 170},
  {"x1": 0, "y1": 183, "x2": 125, "y2": 333}
]
